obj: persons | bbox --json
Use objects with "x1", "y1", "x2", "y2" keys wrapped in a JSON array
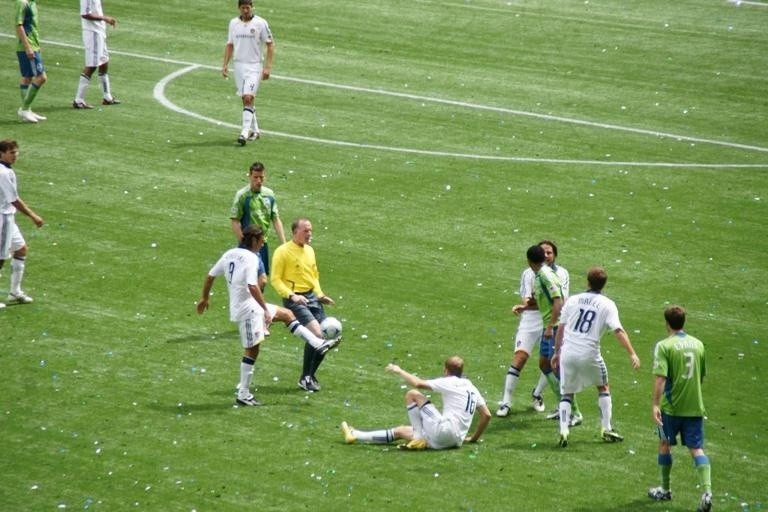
[
  {"x1": 0, "y1": 140, "x2": 45, "y2": 309},
  {"x1": 512, "y1": 246, "x2": 584, "y2": 427},
  {"x1": 551, "y1": 267, "x2": 641, "y2": 448},
  {"x1": 270, "y1": 218, "x2": 336, "y2": 392},
  {"x1": 73, "y1": 0, "x2": 121, "y2": 110},
  {"x1": 197, "y1": 225, "x2": 343, "y2": 408},
  {"x1": 497, "y1": 242, "x2": 570, "y2": 417},
  {"x1": 229, "y1": 162, "x2": 286, "y2": 293},
  {"x1": 15, "y1": 0, "x2": 48, "y2": 124},
  {"x1": 648, "y1": 306, "x2": 713, "y2": 512},
  {"x1": 222, "y1": 0, "x2": 274, "y2": 146},
  {"x1": 341, "y1": 356, "x2": 491, "y2": 450}
]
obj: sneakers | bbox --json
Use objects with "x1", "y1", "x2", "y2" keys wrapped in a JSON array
[
  {"x1": 235, "y1": 393, "x2": 260, "y2": 406},
  {"x1": 568, "y1": 413, "x2": 583, "y2": 427},
  {"x1": 237, "y1": 134, "x2": 246, "y2": 145},
  {"x1": 0, "y1": 302, "x2": 6, "y2": 308},
  {"x1": 698, "y1": 494, "x2": 712, "y2": 510},
  {"x1": 559, "y1": 431, "x2": 569, "y2": 447},
  {"x1": 601, "y1": 429, "x2": 624, "y2": 442},
  {"x1": 546, "y1": 409, "x2": 560, "y2": 419},
  {"x1": 531, "y1": 388, "x2": 545, "y2": 412},
  {"x1": 73, "y1": 99, "x2": 94, "y2": 109},
  {"x1": 316, "y1": 335, "x2": 343, "y2": 355},
  {"x1": 102, "y1": 97, "x2": 120, "y2": 105},
  {"x1": 341, "y1": 420, "x2": 356, "y2": 444},
  {"x1": 648, "y1": 487, "x2": 671, "y2": 500},
  {"x1": 8, "y1": 291, "x2": 32, "y2": 303},
  {"x1": 22, "y1": 111, "x2": 47, "y2": 121},
  {"x1": 298, "y1": 374, "x2": 320, "y2": 391},
  {"x1": 248, "y1": 132, "x2": 260, "y2": 141},
  {"x1": 17, "y1": 107, "x2": 38, "y2": 123},
  {"x1": 396, "y1": 438, "x2": 426, "y2": 450},
  {"x1": 496, "y1": 402, "x2": 511, "y2": 417}
]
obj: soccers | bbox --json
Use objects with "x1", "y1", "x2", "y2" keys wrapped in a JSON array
[{"x1": 319, "y1": 316, "x2": 343, "y2": 340}]
